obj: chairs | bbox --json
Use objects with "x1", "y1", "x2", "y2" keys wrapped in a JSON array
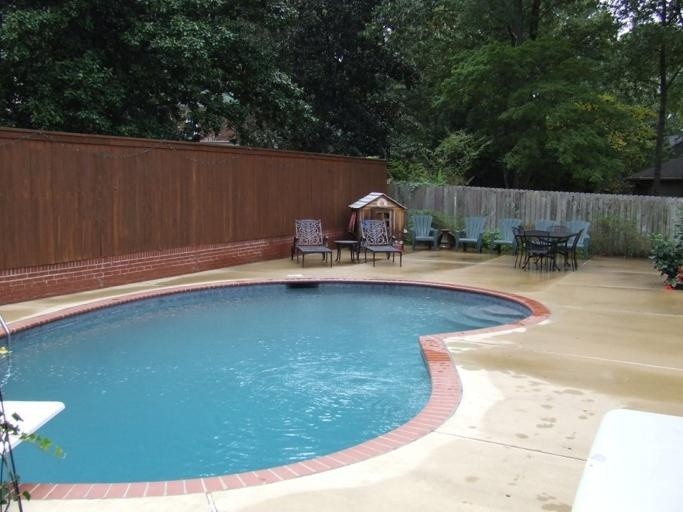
[
  {"x1": 512, "y1": 225, "x2": 585, "y2": 272},
  {"x1": 408, "y1": 215, "x2": 592, "y2": 259},
  {"x1": 289, "y1": 217, "x2": 405, "y2": 268}
]
